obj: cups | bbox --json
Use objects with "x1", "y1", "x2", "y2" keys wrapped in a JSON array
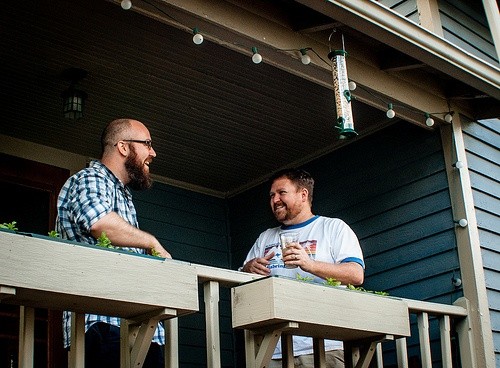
[{"x1": 279, "y1": 231, "x2": 300, "y2": 269}]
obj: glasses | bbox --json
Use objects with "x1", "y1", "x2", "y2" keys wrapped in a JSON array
[{"x1": 114, "y1": 139, "x2": 152, "y2": 150}]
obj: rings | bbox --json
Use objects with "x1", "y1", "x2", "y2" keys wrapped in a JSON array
[{"x1": 295, "y1": 254, "x2": 297, "y2": 260}]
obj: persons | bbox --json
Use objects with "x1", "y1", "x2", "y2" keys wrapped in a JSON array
[
  {"x1": 55, "y1": 118, "x2": 172, "y2": 368},
  {"x1": 236, "y1": 167, "x2": 367, "y2": 368}
]
